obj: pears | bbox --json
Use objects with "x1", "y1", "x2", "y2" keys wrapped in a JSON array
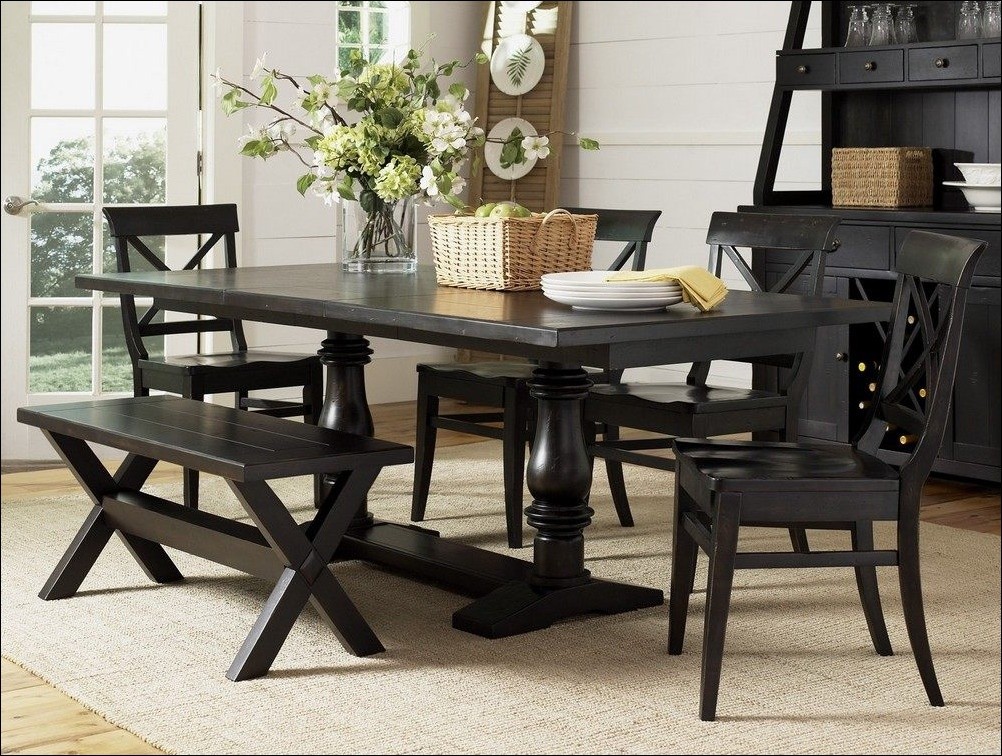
[{"x1": 474, "y1": 197, "x2": 533, "y2": 218}]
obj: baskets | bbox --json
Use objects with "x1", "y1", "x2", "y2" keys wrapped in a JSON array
[
  {"x1": 830, "y1": 147, "x2": 935, "y2": 210},
  {"x1": 426, "y1": 208, "x2": 599, "y2": 290}
]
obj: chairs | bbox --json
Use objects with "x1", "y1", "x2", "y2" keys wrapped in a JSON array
[
  {"x1": 411, "y1": 208, "x2": 661, "y2": 548},
  {"x1": 579, "y1": 210, "x2": 837, "y2": 553},
  {"x1": 665, "y1": 230, "x2": 990, "y2": 722},
  {"x1": 101, "y1": 203, "x2": 323, "y2": 510}
]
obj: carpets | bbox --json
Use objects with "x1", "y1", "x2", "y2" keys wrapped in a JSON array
[{"x1": 1, "y1": 438, "x2": 1001, "y2": 755}]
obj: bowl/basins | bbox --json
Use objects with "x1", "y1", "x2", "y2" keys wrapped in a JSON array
[
  {"x1": 943, "y1": 181, "x2": 1001, "y2": 214},
  {"x1": 952, "y1": 162, "x2": 1001, "y2": 186}
]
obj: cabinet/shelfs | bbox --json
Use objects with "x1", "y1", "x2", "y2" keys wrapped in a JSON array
[{"x1": 751, "y1": 2, "x2": 1002, "y2": 486}]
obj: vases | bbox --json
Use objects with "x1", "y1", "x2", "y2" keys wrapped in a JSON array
[{"x1": 343, "y1": 183, "x2": 421, "y2": 272}]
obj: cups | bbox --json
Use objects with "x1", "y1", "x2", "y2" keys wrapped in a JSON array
[{"x1": 844, "y1": 1, "x2": 1001, "y2": 49}]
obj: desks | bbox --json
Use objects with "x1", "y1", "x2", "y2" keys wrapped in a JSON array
[{"x1": 76, "y1": 261, "x2": 894, "y2": 638}]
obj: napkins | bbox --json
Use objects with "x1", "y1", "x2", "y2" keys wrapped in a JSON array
[{"x1": 607, "y1": 268, "x2": 731, "y2": 313}]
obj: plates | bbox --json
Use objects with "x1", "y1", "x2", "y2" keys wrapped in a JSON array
[{"x1": 540, "y1": 270, "x2": 683, "y2": 311}]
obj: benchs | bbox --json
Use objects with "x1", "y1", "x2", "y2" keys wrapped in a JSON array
[{"x1": 17, "y1": 395, "x2": 415, "y2": 682}]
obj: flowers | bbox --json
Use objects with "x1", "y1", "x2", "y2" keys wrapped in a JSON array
[{"x1": 208, "y1": 30, "x2": 601, "y2": 255}]
